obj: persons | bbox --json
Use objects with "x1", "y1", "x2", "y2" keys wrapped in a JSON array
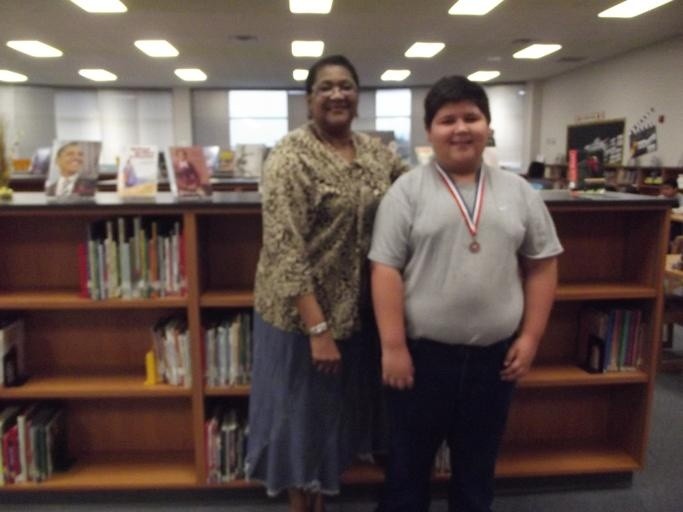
[
  {"x1": 241, "y1": 54, "x2": 420, "y2": 511},
  {"x1": 655, "y1": 177, "x2": 682, "y2": 242},
  {"x1": 365, "y1": 74, "x2": 566, "y2": 511}
]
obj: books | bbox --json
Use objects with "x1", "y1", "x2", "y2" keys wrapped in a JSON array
[
  {"x1": 602, "y1": 169, "x2": 640, "y2": 192},
  {"x1": 78, "y1": 215, "x2": 188, "y2": 300},
  {"x1": 1, "y1": 133, "x2": 263, "y2": 205},
  {"x1": 153, "y1": 309, "x2": 253, "y2": 483},
  {"x1": 573, "y1": 304, "x2": 647, "y2": 375},
  {"x1": 2, "y1": 319, "x2": 68, "y2": 483}
]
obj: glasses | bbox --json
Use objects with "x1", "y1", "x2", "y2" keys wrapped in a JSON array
[{"x1": 310, "y1": 83, "x2": 354, "y2": 99}]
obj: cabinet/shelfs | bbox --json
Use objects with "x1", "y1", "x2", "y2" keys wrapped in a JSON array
[
  {"x1": 1, "y1": 191, "x2": 206, "y2": 495},
  {"x1": 184, "y1": 191, "x2": 281, "y2": 489},
  {"x1": 489, "y1": 187, "x2": 679, "y2": 485}
]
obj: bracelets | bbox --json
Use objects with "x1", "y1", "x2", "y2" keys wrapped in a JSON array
[{"x1": 304, "y1": 322, "x2": 330, "y2": 336}]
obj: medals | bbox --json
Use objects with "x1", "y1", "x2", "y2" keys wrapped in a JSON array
[{"x1": 468, "y1": 241, "x2": 481, "y2": 254}]
